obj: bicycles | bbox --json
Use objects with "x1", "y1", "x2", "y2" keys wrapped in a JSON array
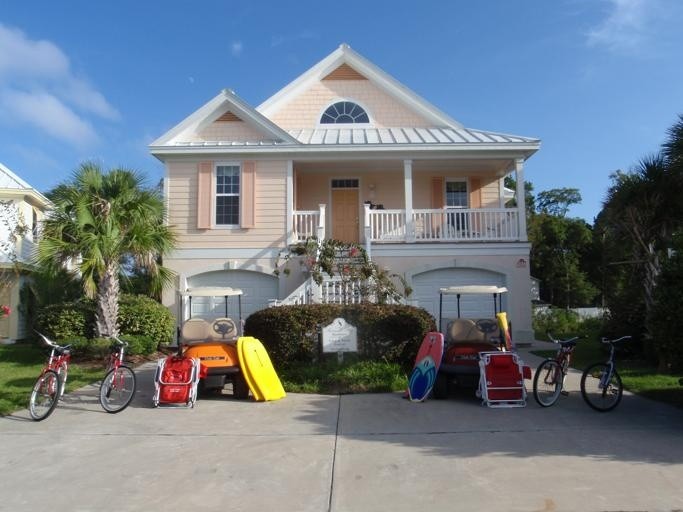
[
  {"x1": 579, "y1": 334, "x2": 636, "y2": 411},
  {"x1": 28, "y1": 328, "x2": 72, "y2": 422},
  {"x1": 532, "y1": 326, "x2": 589, "y2": 407},
  {"x1": 98, "y1": 332, "x2": 136, "y2": 413}
]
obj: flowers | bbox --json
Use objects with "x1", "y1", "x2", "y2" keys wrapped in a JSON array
[
  {"x1": 0, "y1": 304, "x2": 10, "y2": 319},
  {"x1": 274, "y1": 237, "x2": 412, "y2": 306}
]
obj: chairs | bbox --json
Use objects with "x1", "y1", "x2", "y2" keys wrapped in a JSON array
[
  {"x1": 445, "y1": 318, "x2": 500, "y2": 346},
  {"x1": 179, "y1": 317, "x2": 237, "y2": 345}
]
